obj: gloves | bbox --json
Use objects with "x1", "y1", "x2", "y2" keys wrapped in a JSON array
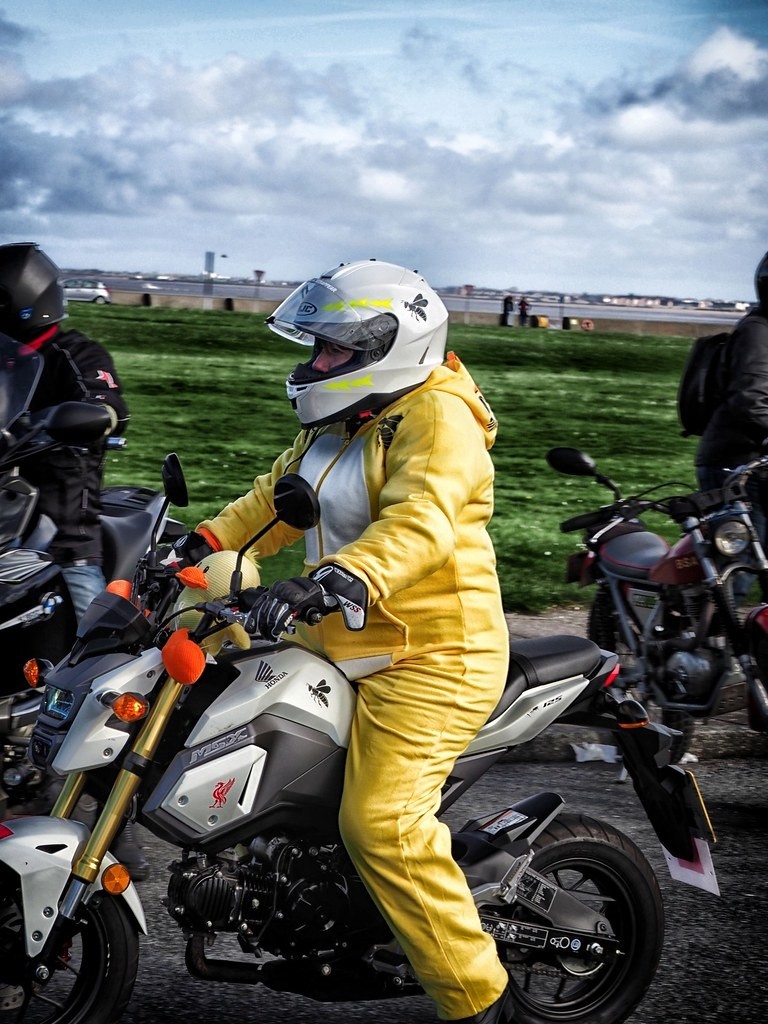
[
  {"x1": 132, "y1": 532, "x2": 213, "y2": 611},
  {"x1": 243, "y1": 562, "x2": 369, "y2": 642}
]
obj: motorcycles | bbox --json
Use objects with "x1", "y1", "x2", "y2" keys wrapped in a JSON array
[
  {"x1": 1, "y1": 456, "x2": 721, "y2": 1024},
  {"x1": 544, "y1": 445, "x2": 768, "y2": 765},
  {"x1": 1, "y1": 401, "x2": 189, "y2": 813}
]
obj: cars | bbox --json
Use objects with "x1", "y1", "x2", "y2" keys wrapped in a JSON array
[{"x1": 58, "y1": 277, "x2": 111, "y2": 305}]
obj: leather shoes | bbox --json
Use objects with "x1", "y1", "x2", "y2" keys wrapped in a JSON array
[
  {"x1": 108, "y1": 816, "x2": 152, "y2": 881},
  {"x1": 464, "y1": 987, "x2": 514, "y2": 1024}
]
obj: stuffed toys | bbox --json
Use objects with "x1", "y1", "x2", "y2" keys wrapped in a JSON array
[{"x1": 162, "y1": 545, "x2": 262, "y2": 684}]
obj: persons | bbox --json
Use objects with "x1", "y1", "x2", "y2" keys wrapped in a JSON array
[
  {"x1": 518, "y1": 296, "x2": 530, "y2": 326},
  {"x1": 503, "y1": 296, "x2": 513, "y2": 327},
  {"x1": 0, "y1": 241, "x2": 130, "y2": 629},
  {"x1": 676, "y1": 252, "x2": 768, "y2": 629},
  {"x1": 152, "y1": 257, "x2": 510, "y2": 1024}
]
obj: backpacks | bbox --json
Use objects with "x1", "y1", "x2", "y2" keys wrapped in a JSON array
[{"x1": 676, "y1": 315, "x2": 768, "y2": 439}]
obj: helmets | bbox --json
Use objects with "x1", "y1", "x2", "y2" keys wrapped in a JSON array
[
  {"x1": 754, "y1": 251, "x2": 768, "y2": 307},
  {"x1": 0, "y1": 241, "x2": 69, "y2": 337},
  {"x1": 285, "y1": 258, "x2": 449, "y2": 430}
]
